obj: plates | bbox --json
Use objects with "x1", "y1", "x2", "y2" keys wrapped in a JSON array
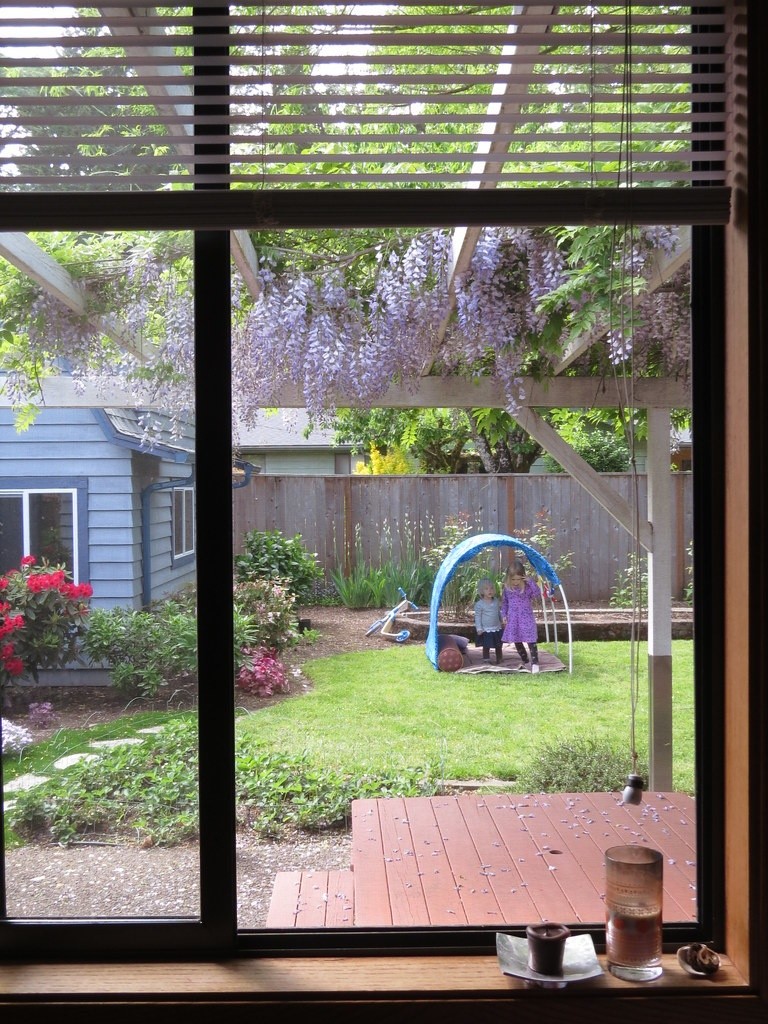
[{"x1": 496, "y1": 932, "x2": 604, "y2": 983}]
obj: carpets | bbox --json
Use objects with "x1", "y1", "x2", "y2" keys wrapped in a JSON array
[{"x1": 455, "y1": 642, "x2": 567, "y2": 675}]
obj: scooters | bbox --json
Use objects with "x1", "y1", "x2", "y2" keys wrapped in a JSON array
[{"x1": 364, "y1": 585, "x2": 419, "y2": 643}]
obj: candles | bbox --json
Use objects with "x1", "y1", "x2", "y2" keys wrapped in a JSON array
[{"x1": 526, "y1": 921, "x2": 570, "y2": 976}]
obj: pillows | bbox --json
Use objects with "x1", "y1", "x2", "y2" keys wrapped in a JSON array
[{"x1": 438, "y1": 634, "x2": 463, "y2": 672}]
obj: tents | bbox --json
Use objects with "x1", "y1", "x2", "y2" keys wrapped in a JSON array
[{"x1": 424, "y1": 534, "x2": 575, "y2": 675}]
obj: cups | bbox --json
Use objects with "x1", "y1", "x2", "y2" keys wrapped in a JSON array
[{"x1": 605, "y1": 846, "x2": 663, "y2": 981}]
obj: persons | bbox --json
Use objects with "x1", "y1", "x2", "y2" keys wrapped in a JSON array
[
  {"x1": 500, "y1": 561, "x2": 541, "y2": 674},
  {"x1": 474, "y1": 580, "x2": 505, "y2": 663}
]
